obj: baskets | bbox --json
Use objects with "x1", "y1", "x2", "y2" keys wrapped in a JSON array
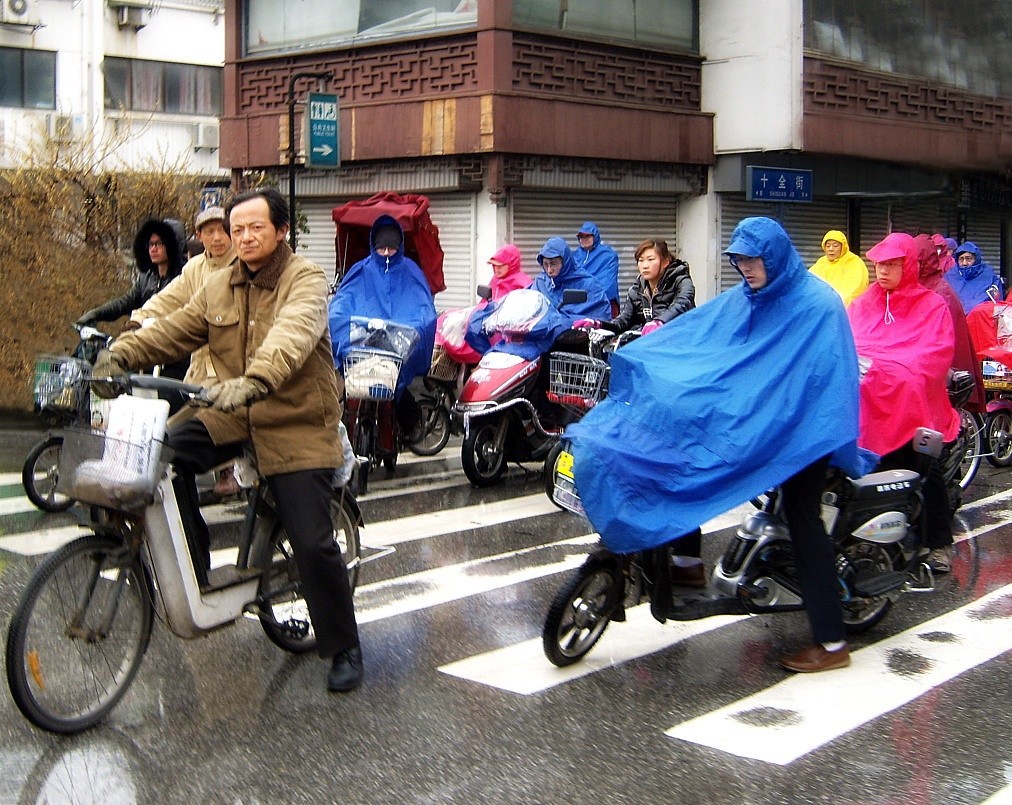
[
  {"x1": 343, "y1": 352, "x2": 402, "y2": 400},
  {"x1": 548, "y1": 352, "x2": 607, "y2": 407},
  {"x1": 551, "y1": 438, "x2": 588, "y2": 518},
  {"x1": 33, "y1": 354, "x2": 90, "y2": 412},
  {"x1": 978, "y1": 353, "x2": 1012, "y2": 391},
  {"x1": 54, "y1": 426, "x2": 176, "y2": 511}
]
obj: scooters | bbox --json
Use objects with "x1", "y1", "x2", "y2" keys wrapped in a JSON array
[
  {"x1": 541, "y1": 273, "x2": 1012, "y2": 668},
  {"x1": 22, "y1": 324, "x2": 118, "y2": 510},
  {"x1": 338, "y1": 286, "x2": 587, "y2": 499}
]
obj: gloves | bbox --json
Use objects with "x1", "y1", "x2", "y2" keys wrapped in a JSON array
[
  {"x1": 206, "y1": 377, "x2": 269, "y2": 413},
  {"x1": 91, "y1": 348, "x2": 130, "y2": 399},
  {"x1": 119, "y1": 321, "x2": 141, "y2": 333},
  {"x1": 573, "y1": 318, "x2": 601, "y2": 331},
  {"x1": 642, "y1": 320, "x2": 663, "y2": 336},
  {"x1": 75, "y1": 312, "x2": 97, "y2": 335}
]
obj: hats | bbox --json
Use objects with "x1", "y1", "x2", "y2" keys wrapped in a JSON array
[
  {"x1": 487, "y1": 259, "x2": 504, "y2": 266},
  {"x1": 195, "y1": 206, "x2": 225, "y2": 230},
  {"x1": 577, "y1": 233, "x2": 592, "y2": 237},
  {"x1": 374, "y1": 226, "x2": 400, "y2": 250}
]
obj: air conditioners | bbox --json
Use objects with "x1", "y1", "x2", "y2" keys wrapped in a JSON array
[
  {"x1": 193, "y1": 124, "x2": 219, "y2": 148},
  {"x1": 118, "y1": 6, "x2": 145, "y2": 26},
  {"x1": 46, "y1": 114, "x2": 82, "y2": 143},
  {"x1": 0, "y1": 0, "x2": 30, "y2": 24}
]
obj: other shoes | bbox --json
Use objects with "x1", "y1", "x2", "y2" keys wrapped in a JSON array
[
  {"x1": 212, "y1": 471, "x2": 240, "y2": 496},
  {"x1": 326, "y1": 641, "x2": 363, "y2": 691},
  {"x1": 410, "y1": 409, "x2": 427, "y2": 442}
]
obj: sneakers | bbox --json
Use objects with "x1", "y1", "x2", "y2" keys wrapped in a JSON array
[
  {"x1": 927, "y1": 546, "x2": 952, "y2": 571},
  {"x1": 670, "y1": 559, "x2": 707, "y2": 589},
  {"x1": 781, "y1": 641, "x2": 852, "y2": 672}
]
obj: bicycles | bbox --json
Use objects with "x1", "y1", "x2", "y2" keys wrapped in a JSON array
[
  {"x1": 543, "y1": 327, "x2": 640, "y2": 512},
  {"x1": 6, "y1": 375, "x2": 397, "y2": 736}
]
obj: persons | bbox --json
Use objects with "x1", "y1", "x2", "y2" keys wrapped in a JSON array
[
  {"x1": 74, "y1": 205, "x2": 238, "y2": 496},
  {"x1": 626, "y1": 217, "x2": 851, "y2": 673},
  {"x1": 801, "y1": 228, "x2": 1012, "y2": 571},
  {"x1": 328, "y1": 213, "x2": 439, "y2": 445},
  {"x1": 477, "y1": 221, "x2": 698, "y2": 363},
  {"x1": 90, "y1": 189, "x2": 364, "y2": 692}
]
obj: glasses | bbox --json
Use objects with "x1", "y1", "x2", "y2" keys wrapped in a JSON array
[
  {"x1": 957, "y1": 255, "x2": 975, "y2": 262},
  {"x1": 874, "y1": 262, "x2": 902, "y2": 267},
  {"x1": 146, "y1": 241, "x2": 164, "y2": 248},
  {"x1": 729, "y1": 255, "x2": 755, "y2": 266}
]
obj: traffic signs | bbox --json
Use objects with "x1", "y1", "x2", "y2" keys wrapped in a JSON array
[{"x1": 306, "y1": 94, "x2": 341, "y2": 170}]
